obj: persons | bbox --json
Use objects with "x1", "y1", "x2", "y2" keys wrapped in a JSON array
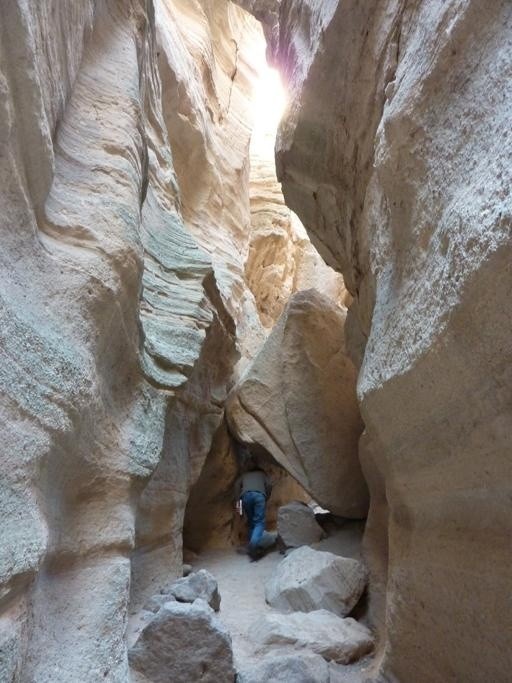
[{"x1": 234, "y1": 466, "x2": 271, "y2": 553}]
